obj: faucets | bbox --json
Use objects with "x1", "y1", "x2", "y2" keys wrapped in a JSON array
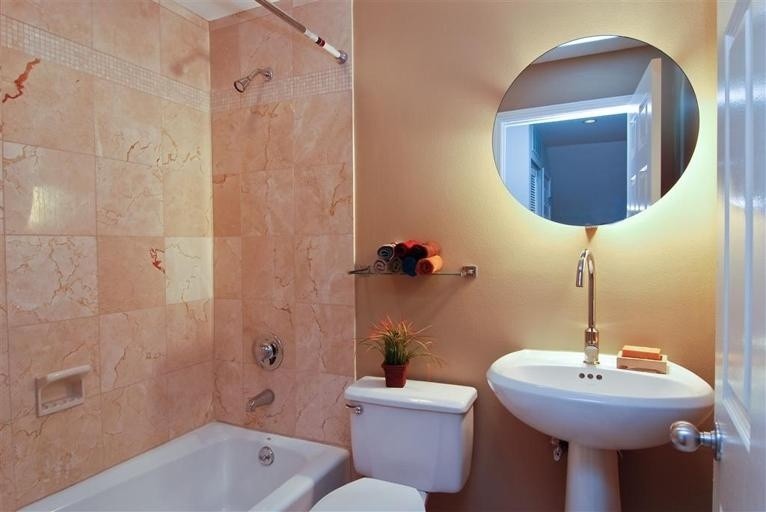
[
  {"x1": 575, "y1": 246, "x2": 601, "y2": 361},
  {"x1": 245, "y1": 389, "x2": 276, "y2": 413}
]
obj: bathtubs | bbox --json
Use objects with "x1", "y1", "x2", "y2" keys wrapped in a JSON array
[{"x1": 14, "y1": 420, "x2": 350, "y2": 511}]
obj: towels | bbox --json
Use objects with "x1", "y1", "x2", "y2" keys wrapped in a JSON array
[{"x1": 374, "y1": 240, "x2": 444, "y2": 277}]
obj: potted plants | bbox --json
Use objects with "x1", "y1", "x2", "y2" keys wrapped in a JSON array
[{"x1": 356, "y1": 311, "x2": 433, "y2": 388}]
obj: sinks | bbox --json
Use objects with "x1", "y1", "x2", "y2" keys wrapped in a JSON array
[{"x1": 486, "y1": 349, "x2": 713, "y2": 452}]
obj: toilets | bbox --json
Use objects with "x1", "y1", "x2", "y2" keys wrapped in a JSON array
[{"x1": 307, "y1": 376, "x2": 477, "y2": 510}]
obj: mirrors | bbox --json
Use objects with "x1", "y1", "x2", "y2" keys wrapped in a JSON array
[{"x1": 491, "y1": 33, "x2": 699, "y2": 228}]
